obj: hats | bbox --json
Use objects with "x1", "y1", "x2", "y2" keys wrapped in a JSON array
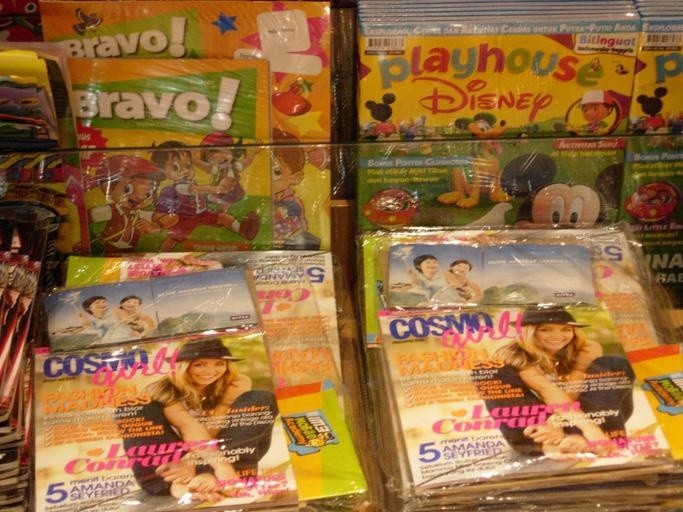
[
  {"x1": 582, "y1": 90, "x2": 614, "y2": 105},
  {"x1": 510, "y1": 303, "x2": 589, "y2": 327},
  {"x1": 199, "y1": 131, "x2": 233, "y2": 159},
  {"x1": 166, "y1": 336, "x2": 245, "y2": 360},
  {"x1": 96, "y1": 155, "x2": 166, "y2": 181}
]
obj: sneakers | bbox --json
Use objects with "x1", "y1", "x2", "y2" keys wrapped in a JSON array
[{"x1": 239, "y1": 211, "x2": 259, "y2": 241}]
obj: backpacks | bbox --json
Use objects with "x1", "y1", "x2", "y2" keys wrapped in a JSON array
[{"x1": 158, "y1": 180, "x2": 190, "y2": 213}]
